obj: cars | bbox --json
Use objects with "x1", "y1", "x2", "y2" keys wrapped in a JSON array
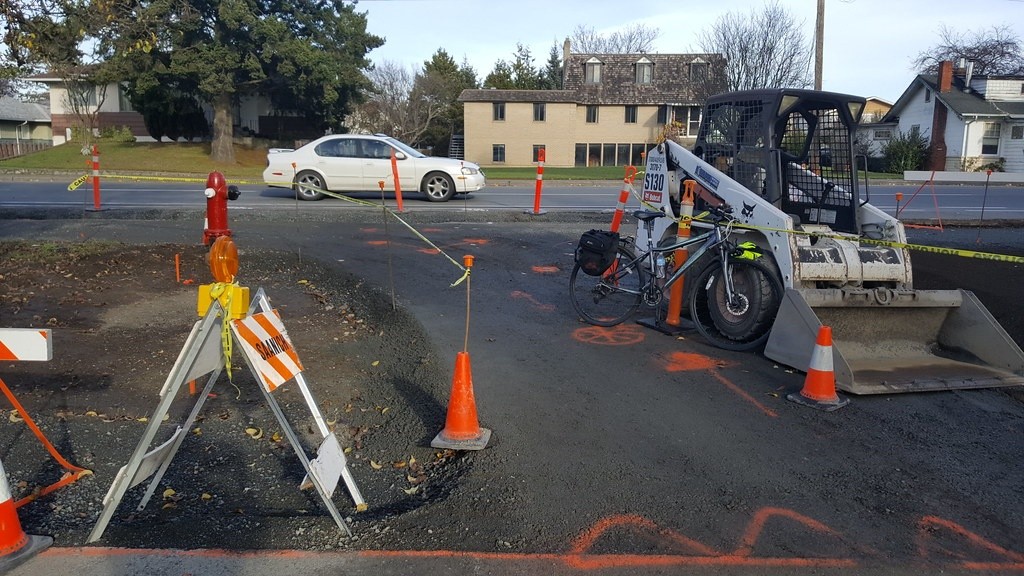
[{"x1": 263, "y1": 134, "x2": 486, "y2": 203}]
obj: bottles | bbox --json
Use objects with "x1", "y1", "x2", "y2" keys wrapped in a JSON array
[{"x1": 656, "y1": 252, "x2": 666, "y2": 279}]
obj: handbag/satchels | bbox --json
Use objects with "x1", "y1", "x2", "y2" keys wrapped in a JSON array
[{"x1": 575, "y1": 229, "x2": 620, "y2": 276}]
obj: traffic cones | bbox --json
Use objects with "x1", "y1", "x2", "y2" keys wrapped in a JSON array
[
  {"x1": 611, "y1": 165, "x2": 637, "y2": 232},
  {"x1": 85, "y1": 149, "x2": 110, "y2": 213},
  {"x1": 524, "y1": 148, "x2": 547, "y2": 216},
  {"x1": 636, "y1": 179, "x2": 699, "y2": 335},
  {"x1": 430, "y1": 351, "x2": 492, "y2": 451},
  {"x1": 389, "y1": 146, "x2": 411, "y2": 215},
  {"x1": 787, "y1": 326, "x2": 851, "y2": 413}
]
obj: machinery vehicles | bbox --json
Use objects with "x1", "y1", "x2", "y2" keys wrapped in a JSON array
[{"x1": 633, "y1": 90, "x2": 1024, "y2": 395}]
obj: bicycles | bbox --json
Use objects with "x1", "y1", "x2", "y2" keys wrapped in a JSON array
[{"x1": 568, "y1": 207, "x2": 782, "y2": 352}]
